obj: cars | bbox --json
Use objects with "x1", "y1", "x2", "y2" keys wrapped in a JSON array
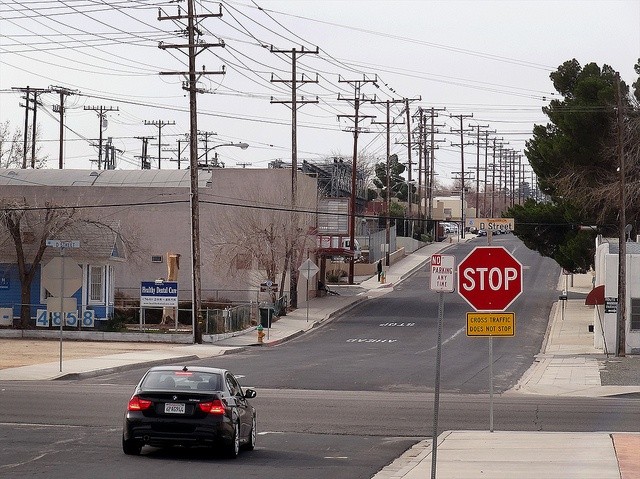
[{"x1": 123, "y1": 365, "x2": 257, "y2": 457}]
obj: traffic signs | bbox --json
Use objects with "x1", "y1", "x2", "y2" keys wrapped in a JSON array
[
  {"x1": 140, "y1": 280, "x2": 178, "y2": 307},
  {"x1": 46, "y1": 240, "x2": 81, "y2": 248},
  {"x1": 466, "y1": 312, "x2": 515, "y2": 337},
  {"x1": 428, "y1": 254, "x2": 456, "y2": 293}
]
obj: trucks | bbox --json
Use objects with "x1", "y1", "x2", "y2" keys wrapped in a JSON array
[
  {"x1": 317, "y1": 237, "x2": 363, "y2": 262},
  {"x1": 466, "y1": 217, "x2": 514, "y2": 236}
]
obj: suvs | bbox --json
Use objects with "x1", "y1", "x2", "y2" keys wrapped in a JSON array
[{"x1": 440, "y1": 222, "x2": 458, "y2": 233}]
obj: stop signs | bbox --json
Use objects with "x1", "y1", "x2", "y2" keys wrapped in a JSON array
[{"x1": 458, "y1": 246, "x2": 523, "y2": 312}]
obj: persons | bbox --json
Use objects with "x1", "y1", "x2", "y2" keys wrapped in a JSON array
[{"x1": 377, "y1": 260, "x2": 383, "y2": 282}]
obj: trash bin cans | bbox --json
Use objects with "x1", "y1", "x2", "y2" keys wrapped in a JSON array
[{"x1": 259, "y1": 307, "x2": 275, "y2": 327}]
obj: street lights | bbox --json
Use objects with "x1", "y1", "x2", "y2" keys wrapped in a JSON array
[
  {"x1": 190, "y1": 143, "x2": 250, "y2": 343},
  {"x1": 385, "y1": 180, "x2": 416, "y2": 265}
]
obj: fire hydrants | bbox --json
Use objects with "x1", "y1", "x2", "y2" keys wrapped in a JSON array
[
  {"x1": 380, "y1": 272, "x2": 385, "y2": 283},
  {"x1": 257, "y1": 324, "x2": 266, "y2": 342}
]
